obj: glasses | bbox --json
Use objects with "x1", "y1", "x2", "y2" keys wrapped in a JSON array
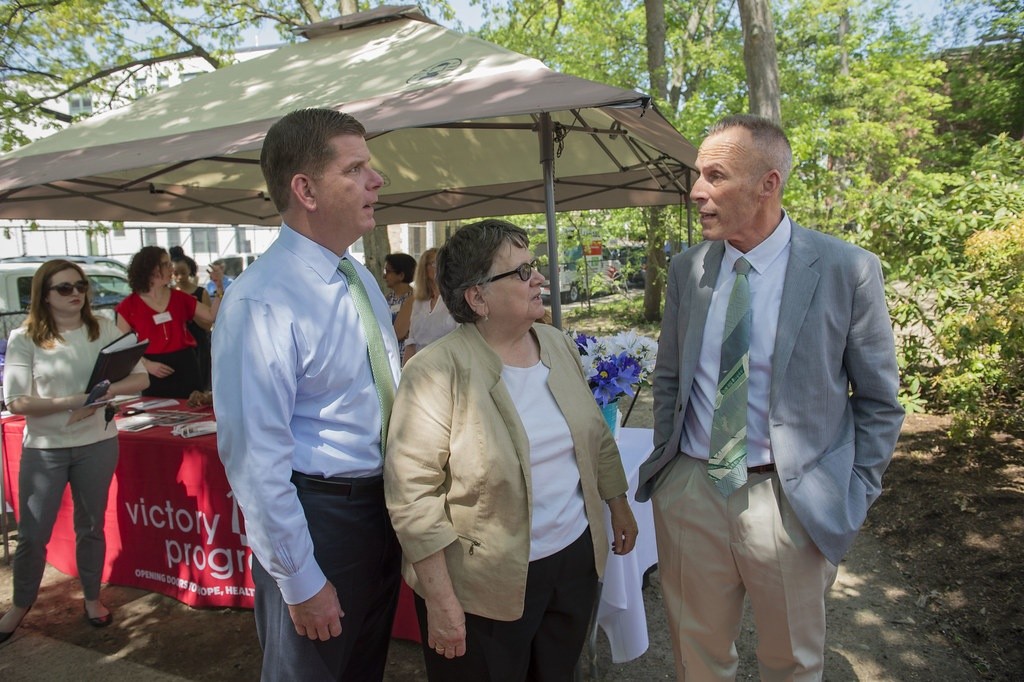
[
  {"x1": 478, "y1": 259, "x2": 539, "y2": 284},
  {"x1": 48, "y1": 280, "x2": 88, "y2": 296}
]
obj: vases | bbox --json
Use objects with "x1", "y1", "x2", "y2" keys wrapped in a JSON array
[{"x1": 600, "y1": 398, "x2": 620, "y2": 438}]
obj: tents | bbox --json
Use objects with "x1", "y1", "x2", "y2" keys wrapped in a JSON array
[{"x1": 0, "y1": 4, "x2": 699, "y2": 331}]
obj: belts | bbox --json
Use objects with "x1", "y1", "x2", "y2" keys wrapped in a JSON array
[
  {"x1": 748, "y1": 463, "x2": 774, "y2": 474},
  {"x1": 291, "y1": 475, "x2": 350, "y2": 496}
]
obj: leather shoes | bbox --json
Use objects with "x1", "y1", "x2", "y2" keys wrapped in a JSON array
[
  {"x1": 0, "y1": 606, "x2": 31, "y2": 643},
  {"x1": 83, "y1": 601, "x2": 112, "y2": 627}
]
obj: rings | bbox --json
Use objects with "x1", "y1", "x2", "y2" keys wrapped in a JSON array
[{"x1": 436, "y1": 646, "x2": 445, "y2": 651}]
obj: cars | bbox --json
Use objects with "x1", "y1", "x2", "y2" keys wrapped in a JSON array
[
  {"x1": 537, "y1": 224, "x2": 693, "y2": 302},
  {"x1": 210, "y1": 251, "x2": 268, "y2": 283},
  {"x1": 0, "y1": 252, "x2": 134, "y2": 340}
]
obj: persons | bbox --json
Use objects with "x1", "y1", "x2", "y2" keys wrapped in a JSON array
[
  {"x1": 211, "y1": 109, "x2": 402, "y2": 682},
  {"x1": 382, "y1": 220, "x2": 639, "y2": 682},
  {"x1": 112, "y1": 245, "x2": 241, "y2": 400},
  {"x1": 0, "y1": 247, "x2": 150, "y2": 644},
  {"x1": 634, "y1": 113, "x2": 905, "y2": 682}
]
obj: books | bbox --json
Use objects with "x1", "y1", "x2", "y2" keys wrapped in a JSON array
[{"x1": 85, "y1": 331, "x2": 150, "y2": 392}]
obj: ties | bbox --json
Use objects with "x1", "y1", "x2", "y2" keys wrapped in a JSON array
[
  {"x1": 338, "y1": 257, "x2": 398, "y2": 461},
  {"x1": 708, "y1": 255, "x2": 751, "y2": 499}
]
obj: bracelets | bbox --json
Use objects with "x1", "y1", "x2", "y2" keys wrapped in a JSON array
[
  {"x1": 217, "y1": 290, "x2": 224, "y2": 300},
  {"x1": 65, "y1": 396, "x2": 72, "y2": 413},
  {"x1": 604, "y1": 492, "x2": 627, "y2": 503},
  {"x1": 108, "y1": 386, "x2": 115, "y2": 398}
]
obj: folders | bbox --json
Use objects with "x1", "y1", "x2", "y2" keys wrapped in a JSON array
[{"x1": 84, "y1": 330, "x2": 150, "y2": 396}]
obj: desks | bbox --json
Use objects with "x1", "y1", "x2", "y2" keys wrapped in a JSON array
[
  {"x1": 574, "y1": 427, "x2": 658, "y2": 682},
  {"x1": 0, "y1": 397, "x2": 422, "y2": 644}
]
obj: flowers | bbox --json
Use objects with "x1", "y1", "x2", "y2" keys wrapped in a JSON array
[{"x1": 561, "y1": 326, "x2": 659, "y2": 408}]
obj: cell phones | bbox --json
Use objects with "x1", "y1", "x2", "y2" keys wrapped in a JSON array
[{"x1": 84, "y1": 380, "x2": 110, "y2": 406}]
